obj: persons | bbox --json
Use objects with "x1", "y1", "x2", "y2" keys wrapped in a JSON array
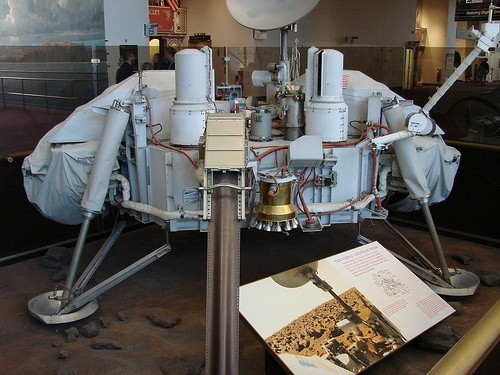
[
  {"x1": 465, "y1": 64, "x2": 474, "y2": 82},
  {"x1": 474, "y1": 64, "x2": 479, "y2": 82},
  {"x1": 153, "y1": 52, "x2": 163, "y2": 70},
  {"x1": 141, "y1": 62, "x2": 152, "y2": 70},
  {"x1": 116, "y1": 50, "x2": 135, "y2": 84},
  {"x1": 161, "y1": 46, "x2": 175, "y2": 70},
  {"x1": 479, "y1": 58, "x2": 489, "y2": 83}
]
